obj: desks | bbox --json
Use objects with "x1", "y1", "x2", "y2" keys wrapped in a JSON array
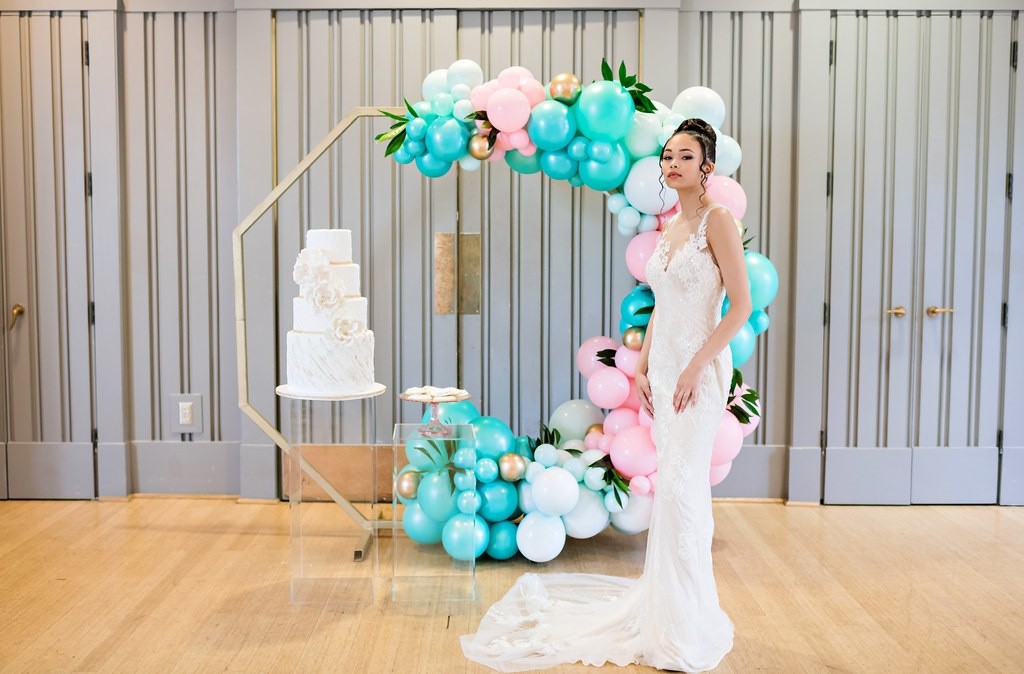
[
  {"x1": 393, "y1": 423, "x2": 475, "y2": 601},
  {"x1": 277, "y1": 383, "x2": 386, "y2": 605}
]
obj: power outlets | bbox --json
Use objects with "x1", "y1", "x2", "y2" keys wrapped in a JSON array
[{"x1": 179, "y1": 402, "x2": 192, "y2": 425}]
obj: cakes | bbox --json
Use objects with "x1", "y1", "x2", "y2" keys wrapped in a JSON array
[{"x1": 286, "y1": 229, "x2": 375, "y2": 394}]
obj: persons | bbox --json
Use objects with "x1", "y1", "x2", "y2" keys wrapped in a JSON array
[{"x1": 461, "y1": 118, "x2": 753, "y2": 673}]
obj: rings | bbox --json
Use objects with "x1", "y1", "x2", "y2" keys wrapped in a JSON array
[{"x1": 684, "y1": 394, "x2": 689, "y2": 397}]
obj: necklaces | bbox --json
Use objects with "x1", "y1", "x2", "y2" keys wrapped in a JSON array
[{"x1": 673, "y1": 201, "x2": 712, "y2": 227}]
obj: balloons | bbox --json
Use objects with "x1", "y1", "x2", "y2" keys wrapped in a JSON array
[{"x1": 392, "y1": 60, "x2": 778, "y2": 562}]
